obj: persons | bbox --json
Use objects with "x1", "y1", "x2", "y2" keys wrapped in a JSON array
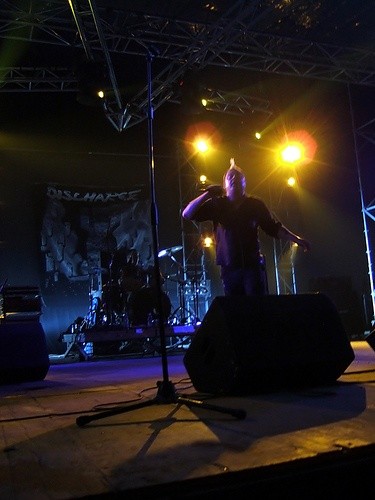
[{"x1": 181, "y1": 164, "x2": 312, "y2": 296}]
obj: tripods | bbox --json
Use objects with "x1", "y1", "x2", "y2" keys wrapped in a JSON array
[
  {"x1": 168, "y1": 256, "x2": 195, "y2": 325},
  {"x1": 76, "y1": 38, "x2": 248, "y2": 427}
]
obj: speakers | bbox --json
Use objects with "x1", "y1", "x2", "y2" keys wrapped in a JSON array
[
  {"x1": 183, "y1": 294, "x2": 355, "y2": 396},
  {"x1": 0, "y1": 321, "x2": 50, "y2": 386}
]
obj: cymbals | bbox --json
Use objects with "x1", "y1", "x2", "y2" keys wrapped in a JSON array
[{"x1": 158, "y1": 246, "x2": 182, "y2": 257}]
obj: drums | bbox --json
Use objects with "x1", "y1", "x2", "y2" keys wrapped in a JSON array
[
  {"x1": 144, "y1": 269, "x2": 160, "y2": 289},
  {"x1": 102, "y1": 286, "x2": 127, "y2": 312},
  {"x1": 121, "y1": 266, "x2": 143, "y2": 290},
  {"x1": 127, "y1": 289, "x2": 171, "y2": 325}
]
